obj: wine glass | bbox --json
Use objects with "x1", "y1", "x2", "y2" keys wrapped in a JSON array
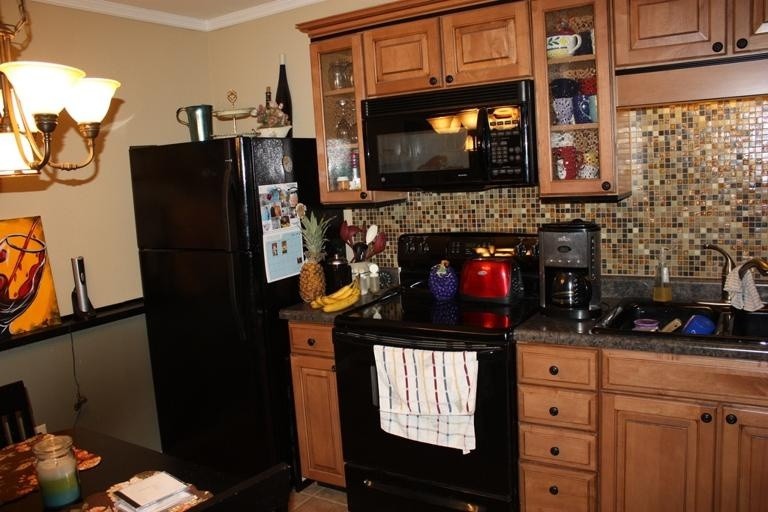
[{"x1": 333, "y1": 98, "x2": 358, "y2": 144}]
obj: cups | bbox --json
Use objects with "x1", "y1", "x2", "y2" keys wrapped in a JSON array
[
  {"x1": 176, "y1": 104, "x2": 213, "y2": 142},
  {"x1": 682, "y1": 314, "x2": 715, "y2": 335},
  {"x1": 326, "y1": 59, "x2": 353, "y2": 90},
  {"x1": 0, "y1": 233, "x2": 48, "y2": 332},
  {"x1": 547, "y1": 76, "x2": 599, "y2": 125},
  {"x1": 552, "y1": 145, "x2": 584, "y2": 180},
  {"x1": 430, "y1": 263, "x2": 459, "y2": 302},
  {"x1": 546, "y1": 24, "x2": 594, "y2": 59},
  {"x1": 352, "y1": 273, "x2": 370, "y2": 295}
]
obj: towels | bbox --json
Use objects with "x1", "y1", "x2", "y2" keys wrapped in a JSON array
[
  {"x1": 370, "y1": 342, "x2": 481, "y2": 456},
  {"x1": 720, "y1": 264, "x2": 765, "y2": 314}
]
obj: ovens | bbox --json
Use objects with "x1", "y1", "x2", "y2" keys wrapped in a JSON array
[{"x1": 332, "y1": 328, "x2": 520, "y2": 512}]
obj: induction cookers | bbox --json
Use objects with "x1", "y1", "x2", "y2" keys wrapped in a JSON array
[{"x1": 337, "y1": 285, "x2": 535, "y2": 336}]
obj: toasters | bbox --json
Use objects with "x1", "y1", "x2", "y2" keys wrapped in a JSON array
[{"x1": 458, "y1": 256, "x2": 525, "y2": 306}]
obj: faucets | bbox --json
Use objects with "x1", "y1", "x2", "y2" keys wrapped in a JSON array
[{"x1": 739, "y1": 256, "x2": 768, "y2": 280}]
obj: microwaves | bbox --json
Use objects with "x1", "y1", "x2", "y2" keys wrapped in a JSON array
[{"x1": 364, "y1": 79, "x2": 538, "y2": 193}]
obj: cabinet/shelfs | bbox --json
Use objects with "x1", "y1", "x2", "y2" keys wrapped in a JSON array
[
  {"x1": 280, "y1": 310, "x2": 345, "y2": 491},
  {"x1": 513, "y1": 328, "x2": 599, "y2": 512},
  {"x1": 358, "y1": 0, "x2": 534, "y2": 101},
  {"x1": 598, "y1": 335, "x2": 767, "y2": 512},
  {"x1": 528, "y1": 0, "x2": 634, "y2": 204},
  {"x1": 294, "y1": 15, "x2": 407, "y2": 206},
  {"x1": 605, "y1": 0, "x2": 767, "y2": 111}
]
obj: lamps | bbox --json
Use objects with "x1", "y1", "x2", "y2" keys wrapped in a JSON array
[
  {"x1": 0, "y1": 0, "x2": 124, "y2": 174},
  {"x1": 427, "y1": 106, "x2": 521, "y2": 156}
]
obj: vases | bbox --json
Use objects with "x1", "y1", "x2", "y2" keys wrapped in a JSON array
[{"x1": 255, "y1": 125, "x2": 292, "y2": 139}]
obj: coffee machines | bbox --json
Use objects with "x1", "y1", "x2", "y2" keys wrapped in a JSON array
[{"x1": 536, "y1": 219, "x2": 605, "y2": 322}]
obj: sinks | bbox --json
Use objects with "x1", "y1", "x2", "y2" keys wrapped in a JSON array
[
  {"x1": 711, "y1": 301, "x2": 767, "y2": 342},
  {"x1": 592, "y1": 297, "x2": 718, "y2": 339}
]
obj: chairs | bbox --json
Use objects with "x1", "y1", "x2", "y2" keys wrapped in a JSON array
[
  {"x1": 0, "y1": 380, "x2": 39, "y2": 449},
  {"x1": 183, "y1": 458, "x2": 295, "y2": 512}
]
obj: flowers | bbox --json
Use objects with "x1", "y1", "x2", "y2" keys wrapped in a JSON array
[{"x1": 254, "y1": 100, "x2": 291, "y2": 129}]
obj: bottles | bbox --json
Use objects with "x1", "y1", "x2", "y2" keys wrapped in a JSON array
[
  {"x1": 263, "y1": 87, "x2": 273, "y2": 112},
  {"x1": 349, "y1": 151, "x2": 361, "y2": 191},
  {"x1": 325, "y1": 253, "x2": 351, "y2": 296},
  {"x1": 652, "y1": 248, "x2": 673, "y2": 302},
  {"x1": 275, "y1": 52, "x2": 294, "y2": 138},
  {"x1": 32, "y1": 434, "x2": 80, "y2": 509},
  {"x1": 337, "y1": 175, "x2": 351, "y2": 191},
  {"x1": 368, "y1": 264, "x2": 380, "y2": 292}
]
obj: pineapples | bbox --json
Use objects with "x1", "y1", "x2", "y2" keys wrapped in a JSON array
[{"x1": 299, "y1": 261, "x2": 327, "y2": 303}]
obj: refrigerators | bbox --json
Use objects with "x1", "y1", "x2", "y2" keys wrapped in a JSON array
[{"x1": 130, "y1": 136, "x2": 321, "y2": 492}]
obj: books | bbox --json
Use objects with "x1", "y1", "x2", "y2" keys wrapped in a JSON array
[{"x1": 113, "y1": 470, "x2": 188, "y2": 512}]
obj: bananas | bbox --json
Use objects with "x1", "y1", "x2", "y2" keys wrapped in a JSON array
[{"x1": 311, "y1": 274, "x2": 361, "y2": 313}]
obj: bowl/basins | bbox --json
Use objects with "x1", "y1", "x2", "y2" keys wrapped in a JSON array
[{"x1": 633, "y1": 318, "x2": 659, "y2": 329}]
